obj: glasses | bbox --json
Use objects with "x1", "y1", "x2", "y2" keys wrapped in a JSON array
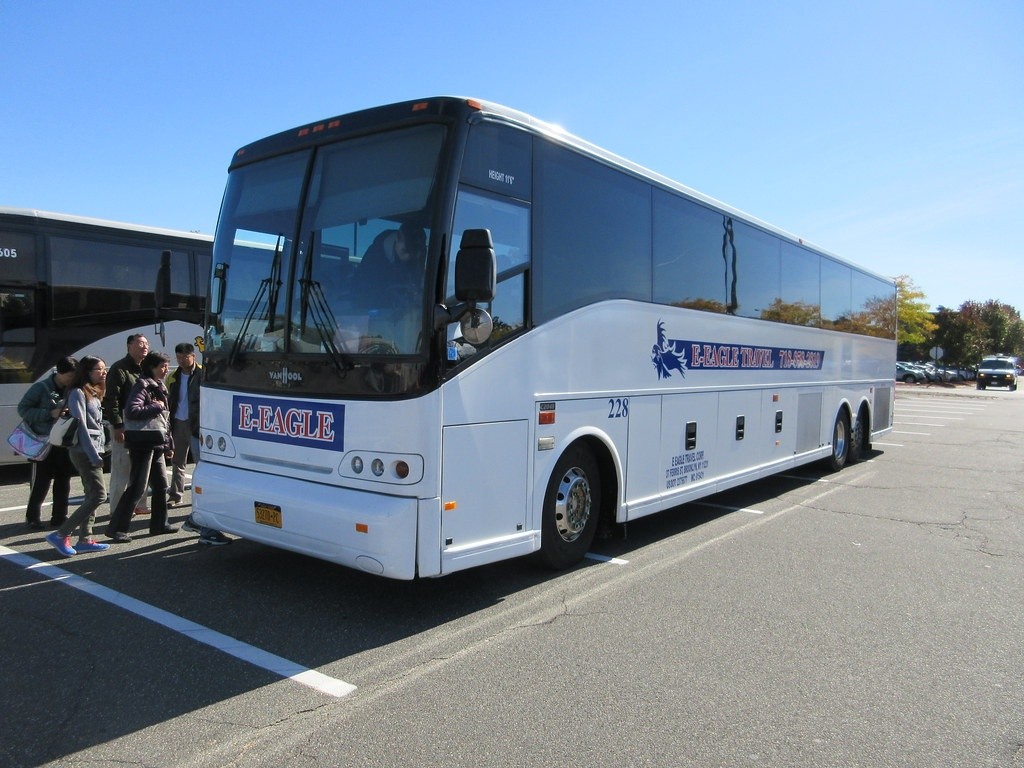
[
  {"x1": 133, "y1": 341, "x2": 150, "y2": 348},
  {"x1": 92, "y1": 367, "x2": 109, "y2": 373},
  {"x1": 175, "y1": 355, "x2": 192, "y2": 360}
]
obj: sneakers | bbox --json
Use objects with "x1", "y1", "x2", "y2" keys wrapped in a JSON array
[
  {"x1": 149, "y1": 525, "x2": 180, "y2": 534},
  {"x1": 181, "y1": 519, "x2": 232, "y2": 545},
  {"x1": 45, "y1": 532, "x2": 77, "y2": 557},
  {"x1": 110, "y1": 507, "x2": 151, "y2": 519},
  {"x1": 105, "y1": 531, "x2": 132, "y2": 543},
  {"x1": 75, "y1": 539, "x2": 111, "y2": 554}
]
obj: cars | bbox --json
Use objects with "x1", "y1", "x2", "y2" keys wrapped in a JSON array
[
  {"x1": 896, "y1": 361, "x2": 977, "y2": 384},
  {"x1": 1016, "y1": 365, "x2": 1023, "y2": 374}
]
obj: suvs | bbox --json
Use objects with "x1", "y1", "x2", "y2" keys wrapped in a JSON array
[{"x1": 976, "y1": 356, "x2": 1018, "y2": 390}]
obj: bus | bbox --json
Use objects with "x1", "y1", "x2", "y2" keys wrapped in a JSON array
[
  {"x1": 152, "y1": 94, "x2": 897, "y2": 582},
  {"x1": 0, "y1": 207, "x2": 365, "y2": 466}
]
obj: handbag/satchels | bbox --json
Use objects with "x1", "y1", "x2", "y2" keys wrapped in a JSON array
[
  {"x1": 48, "y1": 391, "x2": 81, "y2": 449},
  {"x1": 122, "y1": 379, "x2": 171, "y2": 445},
  {"x1": 7, "y1": 418, "x2": 52, "y2": 463}
]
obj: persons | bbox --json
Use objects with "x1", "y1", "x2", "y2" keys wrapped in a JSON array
[
  {"x1": 353, "y1": 222, "x2": 427, "y2": 305},
  {"x1": 18, "y1": 334, "x2": 232, "y2": 557}
]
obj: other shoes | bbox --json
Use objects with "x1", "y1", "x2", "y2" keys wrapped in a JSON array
[
  {"x1": 25, "y1": 517, "x2": 46, "y2": 531},
  {"x1": 166, "y1": 498, "x2": 183, "y2": 504},
  {"x1": 50, "y1": 517, "x2": 69, "y2": 530}
]
